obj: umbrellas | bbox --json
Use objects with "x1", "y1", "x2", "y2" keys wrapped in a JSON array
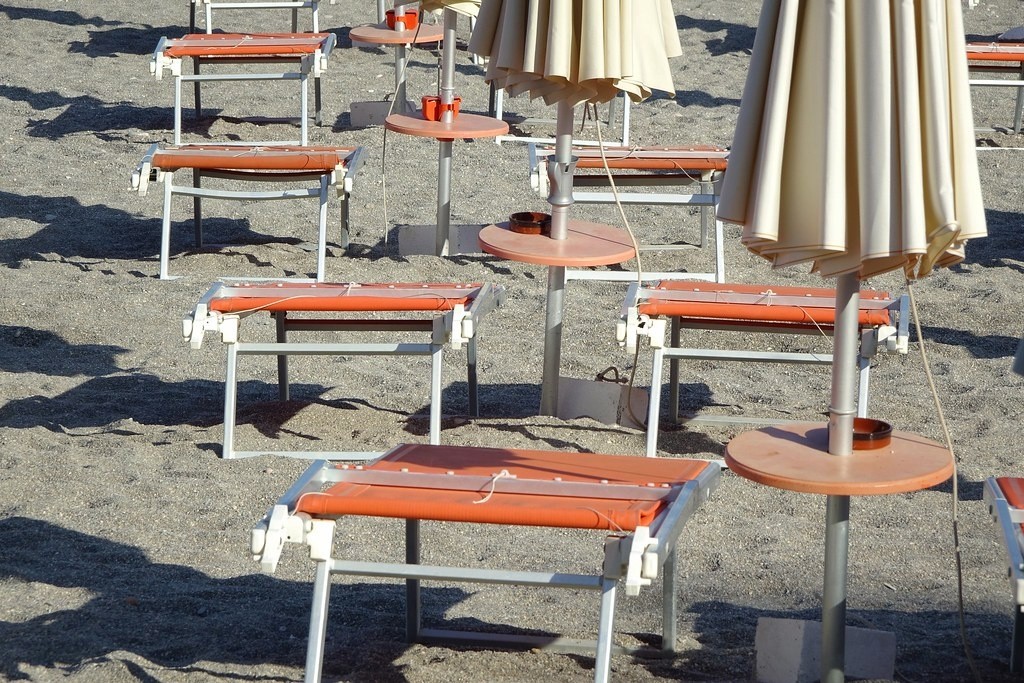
[
  {"x1": 714, "y1": 0, "x2": 988, "y2": 683},
  {"x1": 468, "y1": 0, "x2": 683, "y2": 416},
  {"x1": 393, "y1": 0, "x2": 482, "y2": 255}
]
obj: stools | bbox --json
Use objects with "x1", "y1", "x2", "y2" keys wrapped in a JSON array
[
  {"x1": 525, "y1": 139, "x2": 731, "y2": 284},
  {"x1": 181, "y1": 276, "x2": 508, "y2": 460},
  {"x1": 248, "y1": 443, "x2": 722, "y2": 683},
  {"x1": 130, "y1": 140, "x2": 366, "y2": 282},
  {"x1": 148, "y1": 32, "x2": 340, "y2": 147},
  {"x1": 966, "y1": 39, "x2": 1024, "y2": 152},
  {"x1": 614, "y1": 281, "x2": 908, "y2": 460}
]
